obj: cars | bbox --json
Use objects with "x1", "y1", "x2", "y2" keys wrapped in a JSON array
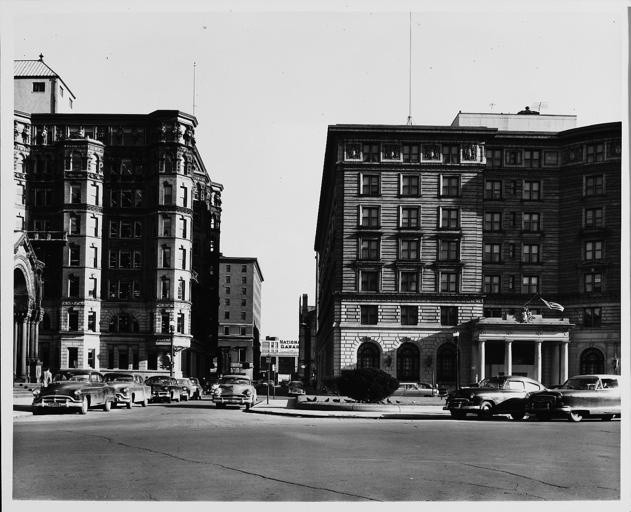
[
  {"x1": 441, "y1": 375, "x2": 546, "y2": 421},
  {"x1": 526, "y1": 374, "x2": 620, "y2": 426},
  {"x1": 391, "y1": 381, "x2": 449, "y2": 399},
  {"x1": 30, "y1": 365, "x2": 308, "y2": 414}
]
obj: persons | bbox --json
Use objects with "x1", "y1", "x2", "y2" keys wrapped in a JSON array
[{"x1": 41, "y1": 367, "x2": 53, "y2": 387}]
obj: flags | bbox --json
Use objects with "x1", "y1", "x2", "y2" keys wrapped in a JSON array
[{"x1": 540, "y1": 298, "x2": 565, "y2": 312}]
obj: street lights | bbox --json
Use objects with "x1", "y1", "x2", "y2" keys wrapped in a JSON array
[
  {"x1": 168, "y1": 320, "x2": 176, "y2": 377},
  {"x1": 452, "y1": 330, "x2": 460, "y2": 390}
]
